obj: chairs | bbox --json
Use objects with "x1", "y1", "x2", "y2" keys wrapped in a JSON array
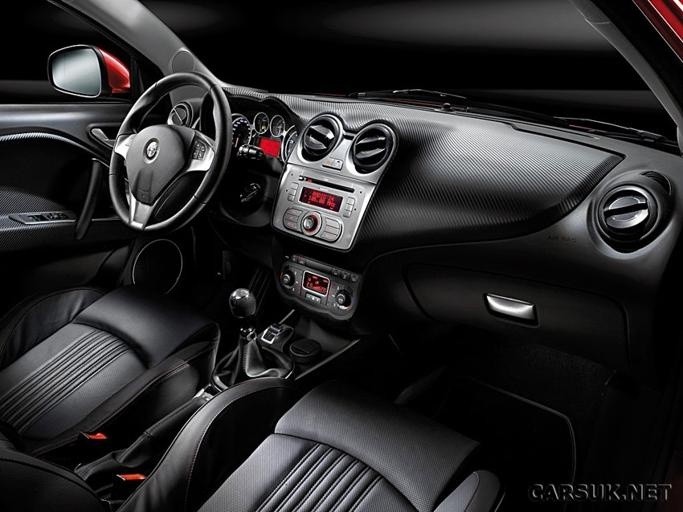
[
  {"x1": 0, "y1": 379, "x2": 507, "y2": 512},
  {"x1": 0, "y1": 285, "x2": 224, "y2": 456}
]
